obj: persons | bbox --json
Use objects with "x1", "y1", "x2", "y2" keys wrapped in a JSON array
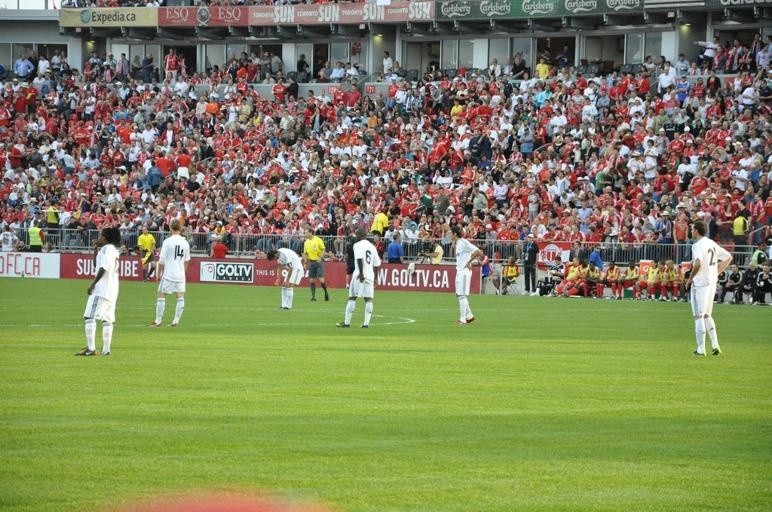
[
  {"x1": 1, "y1": 2, "x2": 771, "y2": 310},
  {"x1": 685, "y1": 222, "x2": 733, "y2": 355},
  {"x1": 76, "y1": 228, "x2": 119, "y2": 356},
  {"x1": 336, "y1": 227, "x2": 381, "y2": 328},
  {"x1": 450, "y1": 226, "x2": 483, "y2": 326},
  {"x1": 150, "y1": 220, "x2": 191, "y2": 326}
]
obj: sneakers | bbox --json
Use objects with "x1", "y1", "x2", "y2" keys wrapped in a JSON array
[
  {"x1": 360, "y1": 324, "x2": 368, "y2": 328},
  {"x1": 74, "y1": 347, "x2": 96, "y2": 356},
  {"x1": 167, "y1": 323, "x2": 176, "y2": 327},
  {"x1": 325, "y1": 295, "x2": 328, "y2": 301},
  {"x1": 311, "y1": 298, "x2": 316, "y2": 301},
  {"x1": 711, "y1": 349, "x2": 720, "y2": 356},
  {"x1": 335, "y1": 321, "x2": 350, "y2": 327},
  {"x1": 456, "y1": 317, "x2": 475, "y2": 324},
  {"x1": 147, "y1": 322, "x2": 161, "y2": 327},
  {"x1": 279, "y1": 307, "x2": 290, "y2": 309},
  {"x1": 693, "y1": 351, "x2": 704, "y2": 356},
  {"x1": 100, "y1": 352, "x2": 108, "y2": 355}
]
